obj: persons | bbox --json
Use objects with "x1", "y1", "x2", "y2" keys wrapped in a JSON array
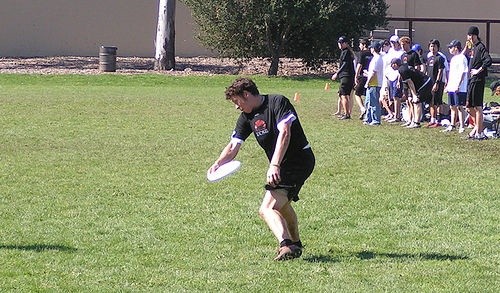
[
  {"x1": 331, "y1": 35, "x2": 476, "y2": 134},
  {"x1": 490, "y1": 80, "x2": 500, "y2": 97},
  {"x1": 206, "y1": 77, "x2": 316, "y2": 262},
  {"x1": 464, "y1": 26, "x2": 493, "y2": 140}
]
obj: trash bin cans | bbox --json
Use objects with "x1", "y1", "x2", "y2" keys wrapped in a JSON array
[{"x1": 99, "y1": 46, "x2": 118, "y2": 72}]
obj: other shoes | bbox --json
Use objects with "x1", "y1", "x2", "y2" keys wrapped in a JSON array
[
  {"x1": 431, "y1": 123, "x2": 440, "y2": 129},
  {"x1": 333, "y1": 111, "x2": 421, "y2": 129},
  {"x1": 459, "y1": 127, "x2": 465, "y2": 133},
  {"x1": 423, "y1": 122, "x2": 435, "y2": 127},
  {"x1": 273, "y1": 245, "x2": 302, "y2": 261},
  {"x1": 467, "y1": 133, "x2": 483, "y2": 140},
  {"x1": 440, "y1": 126, "x2": 453, "y2": 132}
]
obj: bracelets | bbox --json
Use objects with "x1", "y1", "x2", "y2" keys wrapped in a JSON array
[{"x1": 270, "y1": 164, "x2": 280, "y2": 168}]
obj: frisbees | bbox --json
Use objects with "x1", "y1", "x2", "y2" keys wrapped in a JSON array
[{"x1": 207, "y1": 160, "x2": 240, "y2": 183}]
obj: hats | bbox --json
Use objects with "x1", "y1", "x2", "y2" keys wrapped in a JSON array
[
  {"x1": 337, "y1": 36, "x2": 348, "y2": 43},
  {"x1": 468, "y1": 26, "x2": 479, "y2": 35},
  {"x1": 390, "y1": 35, "x2": 398, "y2": 42},
  {"x1": 368, "y1": 42, "x2": 380, "y2": 48},
  {"x1": 446, "y1": 40, "x2": 460, "y2": 47},
  {"x1": 388, "y1": 58, "x2": 401, "y2": 65},
  {"x1": 412, "y1": 44, "x2": 422, "y2": 51}
]
